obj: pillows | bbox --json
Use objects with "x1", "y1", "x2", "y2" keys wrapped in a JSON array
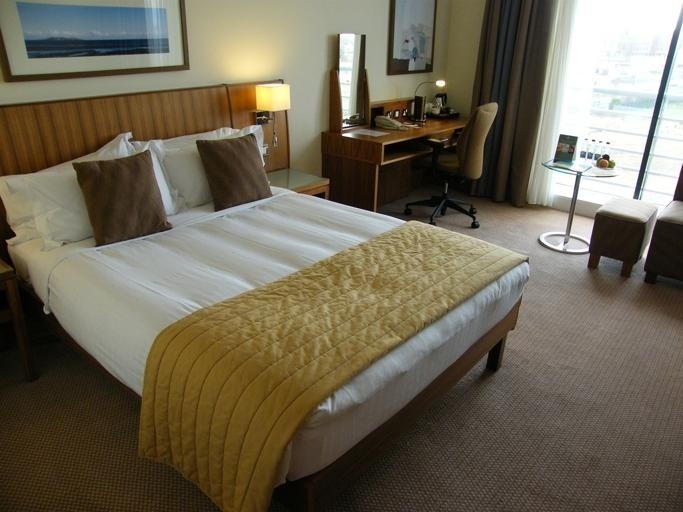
[
  {"x1": 0, "y1": 132, "x2": 133, "y2": 246},
  {"x1": 24, "y1": 141, "x2": 184, "y2": 250},
  {"x1": 196, "y1": 133, "x2": 273, "y2": 211},
  {"x1": 159, "y1": 125, "x2": 263, "y2": 209},
  {"x1": 72, "y1": 150, "x2": 172, "y2": 247}
]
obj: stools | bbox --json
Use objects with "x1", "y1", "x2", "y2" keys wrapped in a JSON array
[{"x1": 586, "y1": 196, "x2": 659, "y2": 278}]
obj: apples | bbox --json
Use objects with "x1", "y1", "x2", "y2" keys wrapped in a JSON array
[{"x1": 596, "y1": 153, "x2": 616, "y2": 169}]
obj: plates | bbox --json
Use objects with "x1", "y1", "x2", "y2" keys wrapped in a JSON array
[{"x1": 592, "y1": 161, "x2": 617, "y2": 171}]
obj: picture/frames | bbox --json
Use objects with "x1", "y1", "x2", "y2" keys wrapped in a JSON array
[
  {"x1": 387, "y1": 0, "x2": 437, "y2": 76},
  {"x1": 0, "y1": 0, "x2": 190, "y2": 82}
]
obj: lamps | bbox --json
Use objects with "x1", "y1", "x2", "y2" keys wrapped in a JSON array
[
  {"x1": 414, "y1": 80, "x2": 446, "y2": 96},
  {"x1": 252, "y1": 83, "x2": 290, "y2": 148}
]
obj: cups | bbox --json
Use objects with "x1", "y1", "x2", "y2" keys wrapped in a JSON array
[{"x1": 432, "y1": 103, "x2": 441, "y2": 115}]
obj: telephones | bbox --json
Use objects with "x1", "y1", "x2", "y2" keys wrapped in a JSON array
[{"x1": 374, "y1": 115, "x2": 403, "y2": 130}]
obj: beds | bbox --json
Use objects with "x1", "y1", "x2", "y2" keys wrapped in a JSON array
[{"x1": 1, "y1": 79, "x2": 528, "y2": 511}]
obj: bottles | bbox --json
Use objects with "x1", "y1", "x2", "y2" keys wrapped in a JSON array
[{"x1": 580, "y1": 138, "x2": 610, "y2": 167}]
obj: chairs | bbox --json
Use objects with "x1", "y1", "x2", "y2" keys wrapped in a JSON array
[
  {"x1": 644, "y1": 164, "x2": 683, "y2": 286},
  {"x1": 404, "y1": 102, "x2": 498, "y2": 229}
]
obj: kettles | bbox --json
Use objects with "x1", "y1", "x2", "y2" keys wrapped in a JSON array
[{"x1": 433, "y1": 93, "x2": 449, "y2": 113}]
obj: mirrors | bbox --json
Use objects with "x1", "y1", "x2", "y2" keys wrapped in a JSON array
[{"x1": 337, "y1": 33, "x2": 366, "y2": 129}]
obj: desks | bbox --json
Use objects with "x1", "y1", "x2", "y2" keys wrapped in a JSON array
[
  {"x1": 267, "y1": 168, "x2": 330, "y2": 200},
  {"x1": 0, "y1": 261, "x2": 37, "y2": 382},
  {"x1": 538, "y1": 159, "x2": 619, "y2": 255},
  {"x1": 322, "y1": 70, "x2": 468, "y2": 213}
]
